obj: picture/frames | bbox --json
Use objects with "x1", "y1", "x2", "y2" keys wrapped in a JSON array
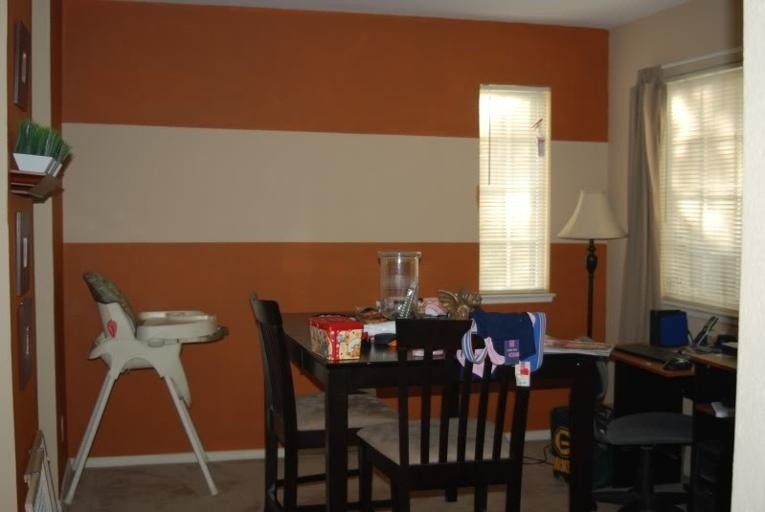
[{"x1": 22, "y1": 432, "x2": 62, "y2": 512}]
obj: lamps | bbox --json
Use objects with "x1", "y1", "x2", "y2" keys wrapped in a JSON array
[{"x1": 556, "y1": 189, "x2": 628, "y2": 341}]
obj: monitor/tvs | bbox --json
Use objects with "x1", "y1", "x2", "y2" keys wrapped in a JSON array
[{"x1": 691, "y1": 315, "x2": 718, "y2": 347}]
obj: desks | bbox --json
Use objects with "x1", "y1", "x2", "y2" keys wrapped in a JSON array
[
  {"x1": 693, "y1": 353, "x2": 737, "y2": 512},
  {"x1": 612, "y1": 346, "x2": 692, "y2": 512}
]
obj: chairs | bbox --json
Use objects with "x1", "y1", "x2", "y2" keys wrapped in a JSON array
[
  {"x1": 64, "y1": 272, "x2": 228, "y2": 507},
  {"x1": 357, "y1": 311, "x2": 541, "y2": 512},
  {"x1": 580, "y1": 360, "x2": 694, "y2": 512},
  {"x1": 247, "y1": 291, "x2": 398, "y2": 512}
]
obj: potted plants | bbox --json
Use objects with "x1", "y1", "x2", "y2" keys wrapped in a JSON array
[{"x1": 13, "y1": 119, "x2": 68, "y2": 174}]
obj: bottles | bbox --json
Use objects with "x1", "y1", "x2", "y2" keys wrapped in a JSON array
[{"x1": 380, "y1": 250, "x2": 423, "y2": 322}]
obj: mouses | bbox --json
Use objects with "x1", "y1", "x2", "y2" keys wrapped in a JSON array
[{"x1": 663, "y1": 356, "x2": 690, "y2": 369}]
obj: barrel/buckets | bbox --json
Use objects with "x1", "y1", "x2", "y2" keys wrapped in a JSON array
[{"x1": 378, "y1": 249, "x2": 422, "y2": 309}]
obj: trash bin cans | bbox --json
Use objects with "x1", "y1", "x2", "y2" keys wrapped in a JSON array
[{"x1": 550, "y1": 406, "x2": 572, "y2": 483}]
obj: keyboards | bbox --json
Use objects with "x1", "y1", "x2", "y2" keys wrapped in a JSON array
[{"x1": 615, "y1": 342, "x2": 694, "y2": 364}]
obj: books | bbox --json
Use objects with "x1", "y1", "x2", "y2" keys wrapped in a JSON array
[{"x1": 543, "y1": 338, "x2": 614, "y2": 357}]
